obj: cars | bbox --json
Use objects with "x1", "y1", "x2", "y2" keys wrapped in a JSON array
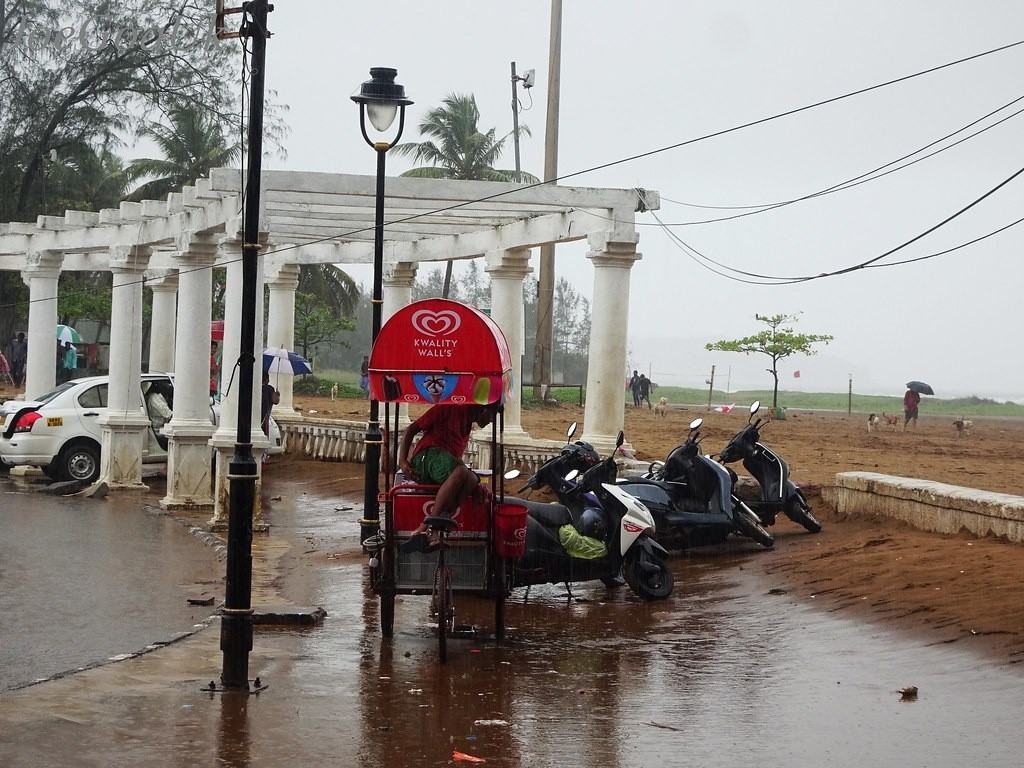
[{"x1": 0, "y1": 370, "x2": 285, "y2": 483}]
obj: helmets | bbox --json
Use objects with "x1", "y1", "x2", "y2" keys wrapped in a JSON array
[{"x1": 560, "y1": 440, "x2": 600, "y2": 473}]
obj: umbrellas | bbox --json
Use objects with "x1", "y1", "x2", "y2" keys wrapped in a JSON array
[
  {"x1": 212, "y1": 321, "x2": 223, "y2": 340},
  {"x1": 57, "y1": 325, "x2": 80, "y2": 343},
  {"x1": 906, "y1": 381, "x2": 934, "y2": 395},
  {"x1": 263, "y1": 343, "x2": 313, "y2": 392}
]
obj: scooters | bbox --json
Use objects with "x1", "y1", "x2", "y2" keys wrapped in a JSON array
[
  {"x1": 701, "y1": 400, "x2": 823, "y2": 536},
  {"x1": 492, "y1": 418, "x2": 675, "y2": 602},
  {"x1": 604, "y1": 417, "x2": 777, "y2": 552}
]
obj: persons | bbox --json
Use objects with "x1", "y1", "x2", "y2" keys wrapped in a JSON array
[
  {"x1": 57, "y1": 340, "x2": 76, "y2": 385},
  {"x1": 398, "y1": 396, "x2": 504, "y2": 556},
  {"x1": 262, "y1": 373, "x2": 280, "y2": 471},
  {"x1": 360, "y1": 356, "x2": 370, "y2": 398},
  {"x1": 902, "y1": 389, "x2": 920, "y2": 433},
  {"x1": 146, "y1": 383, "x2": 173, "y2": 437},
  {"x1": 210, "y1": 341, "x2": 221, "y2": 403},
  {"x1": 628, "y1": 370, "x2": 652, "y2": 409},
  {"x1": 9, "y1": 333, "x2": 26, "y2": 388}
]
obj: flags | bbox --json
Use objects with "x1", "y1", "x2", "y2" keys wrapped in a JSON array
[{"x1": 715, "y1": 403, "x2": 735, "y2": 413}]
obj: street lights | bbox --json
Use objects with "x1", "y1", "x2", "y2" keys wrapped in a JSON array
[{"x1": 347, "y1": 65, "x2": 415, "y2": 548}]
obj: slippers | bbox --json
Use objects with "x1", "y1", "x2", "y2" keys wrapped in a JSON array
[
  {"x1": 399, "y1": 531, "x2": 429, "y2": 554},
  {"x1": 419, "y1": 537, "x2": 442, "y2": 553}
]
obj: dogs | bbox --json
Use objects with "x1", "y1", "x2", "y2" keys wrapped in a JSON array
[
  {"x1": 867, "y1": 413, "x2": 880, "y2": 432},
  {"x1": 953, "y1": 416, "x2": 972, "y2": 437},
  {"x1": 882, "y1": 411, "x2": 901, "y2": 432},
  {"x1": 330, "y1": 383, "x2": 339, "y2": 401},
  {"x1": 653, "y1": 404, "x2": 669, "y2": 418}
]
